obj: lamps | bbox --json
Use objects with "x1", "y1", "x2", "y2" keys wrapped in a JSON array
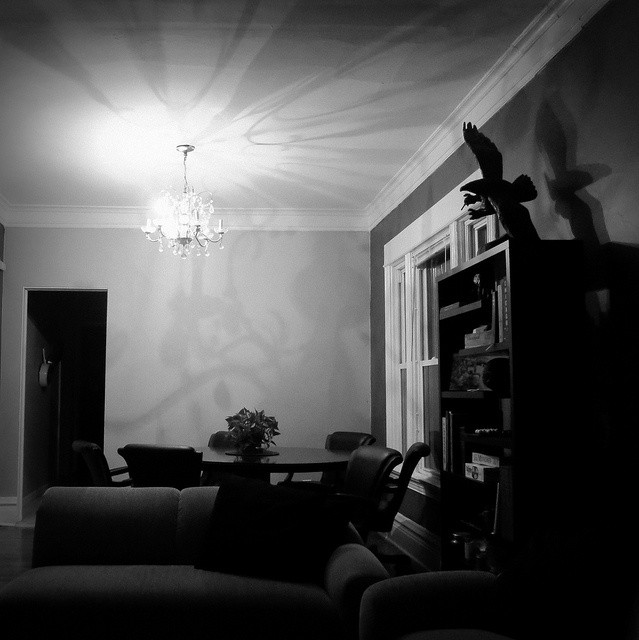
[
  {"x1": 345, "y1": 444, "x2": 403, "y2": 507},
  {"x1": 371, "y1": 442, "x2": 432, "y2": 532},
  {"x1": 320, "y1": 431, "x2": 376, "y2": 483},
  {"x1": 117, "y1": 443, "x2": 204, "y2": 487},
  {"x1": 47, "y1": 439, "x2": 113, "y2": 487},
  {"x1": 208, "y1": 430, "x2": 240, "y2": 449}
]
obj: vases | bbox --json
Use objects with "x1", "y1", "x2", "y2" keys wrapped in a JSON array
[{"x1": 224, "y1": 406, "x2": 282, "y2": 458}]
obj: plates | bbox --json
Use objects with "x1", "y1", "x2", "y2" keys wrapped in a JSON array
[{"x1": 436, "y1": 238, "x2": 638, "y2": 640}]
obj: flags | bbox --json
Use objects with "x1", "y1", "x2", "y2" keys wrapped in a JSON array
[{"x1": 225, "y1": 448, "x2": 280, "y2": 464}]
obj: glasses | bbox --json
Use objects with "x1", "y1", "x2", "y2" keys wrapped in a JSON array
[{"x1": 141, "y1": 145, "x2": 228, "y2": 261}]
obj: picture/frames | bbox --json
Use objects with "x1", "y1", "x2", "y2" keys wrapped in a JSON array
[{"x1": 195, "y1": 445, "x2": 355, "y2": 485}]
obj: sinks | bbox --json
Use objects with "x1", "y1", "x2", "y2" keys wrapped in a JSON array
[
  {"x1": 0, "y1": 484, "x2": 388, "y2": 640},
  {"x1": 359, "y1": 569, "x2": 500, "y2": 640}
]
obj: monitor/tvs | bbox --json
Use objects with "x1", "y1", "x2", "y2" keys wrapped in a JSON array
[
  {"x1": 441, "y1": 411, "x2": 458, "y2": 474},
  {"x1": 491, "y1": 277, "x2": 508, "y2": 343}
]
